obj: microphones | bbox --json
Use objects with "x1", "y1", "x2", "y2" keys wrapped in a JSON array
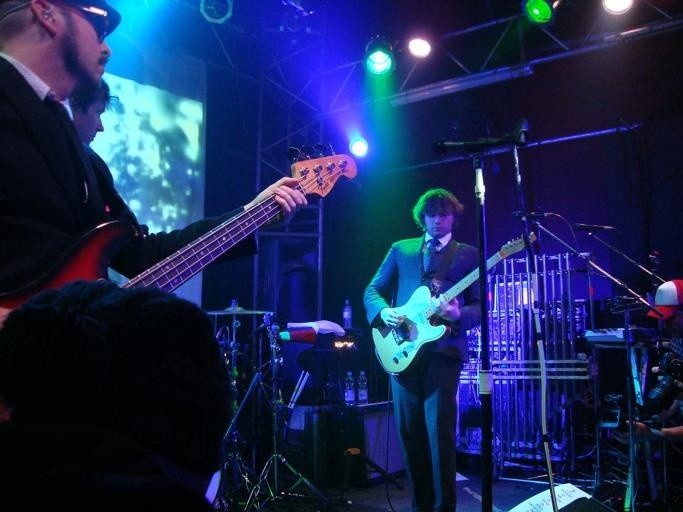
[
  {"x1": 571, "y1": 221, "x2": 615, "y2": 236},
  {"x1": 433, "y1": 136, "x2": 504, "y2": 154}
]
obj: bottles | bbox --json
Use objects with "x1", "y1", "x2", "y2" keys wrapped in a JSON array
[
  {"x1": 341, "y1": 298, "x2": 353, "y2": 328},
  {"x1": 344, "y1": 371, "x2": 355, "y2": 407},
  {"x1": 356, "y1": 370, "x2": 369, "y2": 404}
]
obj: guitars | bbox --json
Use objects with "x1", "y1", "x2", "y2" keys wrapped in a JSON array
[
  {"x1": 371, "y1": 232, "x2": 537, "y2": 379},
  {"x1": 0, "y1": 142, "x2": 358, "y2": 423}
]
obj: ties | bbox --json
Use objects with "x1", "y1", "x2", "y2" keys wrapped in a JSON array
[{"x1": 424, "y1": 239, "x2": 437, "y2": 270}]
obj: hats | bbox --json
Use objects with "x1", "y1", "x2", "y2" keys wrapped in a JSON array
[{"x1": 646, "y1": 280, "x2": 683, "y2": 320}]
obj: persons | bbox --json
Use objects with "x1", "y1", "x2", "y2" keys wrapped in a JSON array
[
  {"x1": 67, "y1": 68, "x2": 110, "y2": 145},
  {"x1": 613, "y1": 278, "x2": 682, "y2": 439},
  {"x1": 0, "y1": 279, "x2": 234, "y2": 511},
  {"x1": 362, "y1": 187, "x2": 491, "y2": 511},
  {"x1": 0, "y1": 2, "x2": 306, "y2": 315}
]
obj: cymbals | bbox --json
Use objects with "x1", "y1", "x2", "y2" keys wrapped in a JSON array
[{"x1": 205, "y1": 306, "x2": 271, "y2": 316}]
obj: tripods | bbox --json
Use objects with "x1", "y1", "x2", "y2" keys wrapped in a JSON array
[{"x1": 221, "y1": 333, "x2": 332, "y2": 512}]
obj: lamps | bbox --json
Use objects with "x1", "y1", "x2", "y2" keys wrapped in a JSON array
[
  {"x1": 522, "y1": 0, "x2": 565, "y2": 26},
  {"x1": 361, "y1": 33, "x2": 397, "y2": 78}
]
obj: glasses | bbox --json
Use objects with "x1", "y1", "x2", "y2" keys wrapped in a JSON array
[{"x1": 73, "y1": 2, "x2": 108, "y2": 41}]
